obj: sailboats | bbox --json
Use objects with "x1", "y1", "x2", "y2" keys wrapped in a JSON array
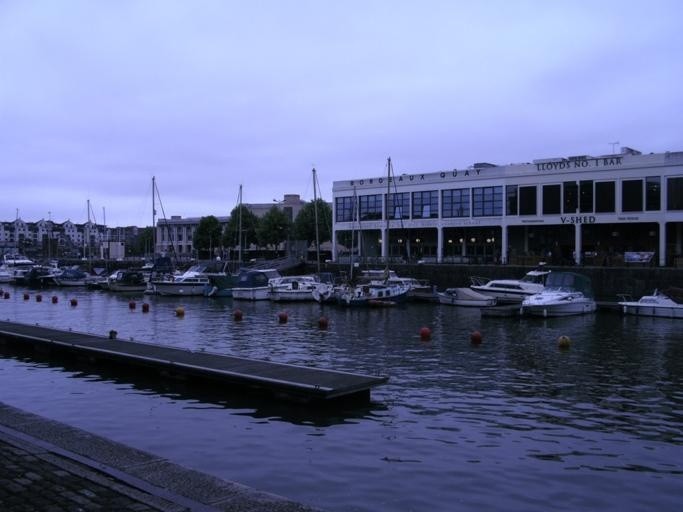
[
  {"x1": 224, "y1": 183, "x2": 283, "y2": 302},
  {"x1": 0, "y1": 199, "x2": 113, "y2": 291},
  {"x1": 266, "y1": 156, "x2": 411, "y2": 308},
  {"x1": 150, "y1": 176, "x2": 211, "y2": 298}
]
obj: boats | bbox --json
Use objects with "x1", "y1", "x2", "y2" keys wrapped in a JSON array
[
  {"x1": 107, "y1": 270, "x2": 148, "y2": 292},
  {"x1": 415, "y1": 261, "x2": 683, "y2": 318}
]
